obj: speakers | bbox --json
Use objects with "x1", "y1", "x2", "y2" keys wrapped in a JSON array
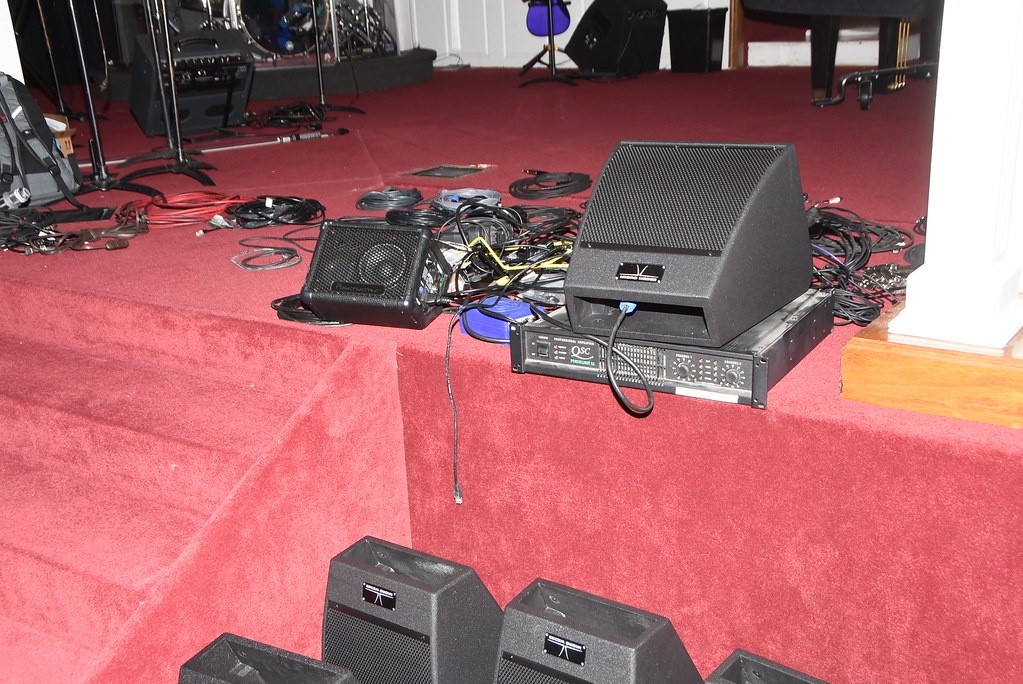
[
  {"x1": 564, "y1": 141, "x2": 813, "y2": 348},
  {"x1": 130, "y1": 28, "x2": 253, "y2": 138},
  {"x1": 178, "y1": 535, "x2": 831, "y2": 684},
  {"x1": 300, "y1": 218, "x2": 454, "y2": 330},
  {"x1": 564, "y1": 0, "x2": 668, "y2": 77}
]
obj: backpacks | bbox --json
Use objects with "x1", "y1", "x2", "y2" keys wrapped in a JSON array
[{"x1": 0, "y1": 71, "x2": 89, "y2": 213}]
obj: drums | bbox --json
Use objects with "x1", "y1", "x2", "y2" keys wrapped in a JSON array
[{"x1": 235, "y1": 0, "x2": 331, "y2": 59}]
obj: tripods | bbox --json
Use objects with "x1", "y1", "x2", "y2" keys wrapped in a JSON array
[
  {"x1": 63, "y1": 0, "x2": 218, "y2": 204},
  {"x1": 297, "y1": 0, "x2": 399, "y2": 114},
  {"x1": 517, "y1": 0, "x2": 587, "y2": 87}
]
